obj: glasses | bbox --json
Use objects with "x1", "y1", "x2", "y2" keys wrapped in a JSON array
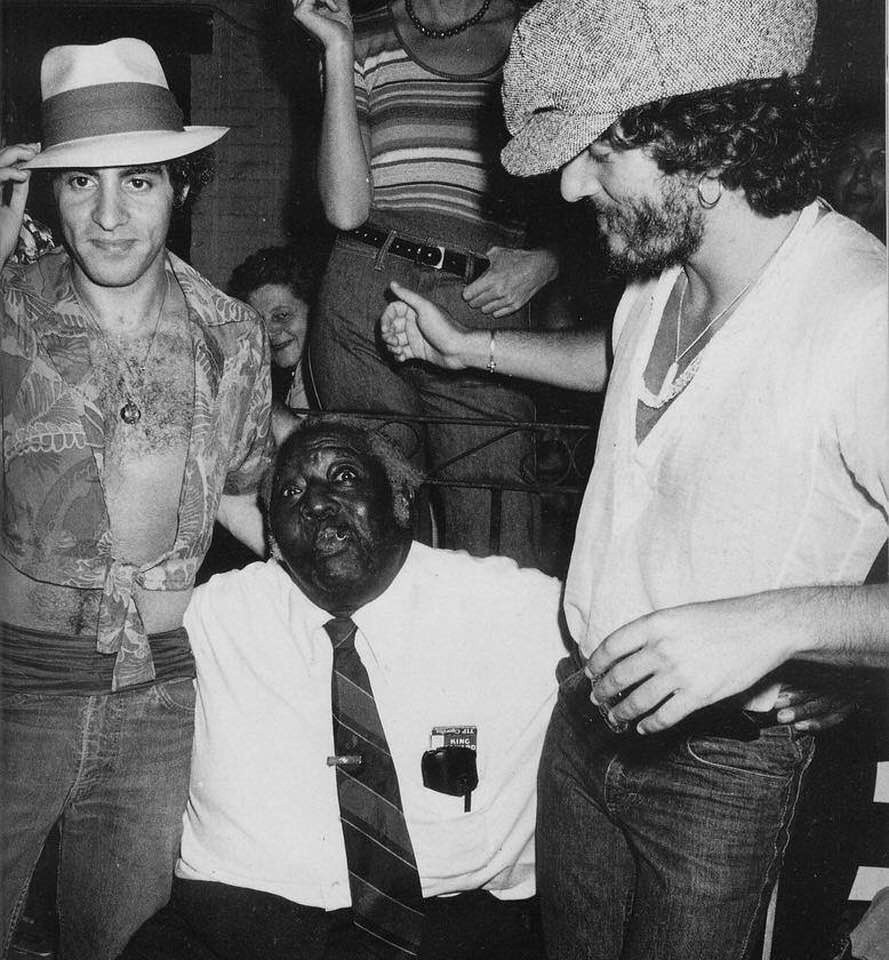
[{"x1": 421, "y1": 745, "x2": 479, "y2": 798}]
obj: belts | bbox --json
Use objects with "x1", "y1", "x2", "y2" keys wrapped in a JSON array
[
  {"x1": 708, "y1": 710, "x2": 778, "y2": 742},
  {"x1": 338, "y1": 225, "x2": 490, "y2": 280}
]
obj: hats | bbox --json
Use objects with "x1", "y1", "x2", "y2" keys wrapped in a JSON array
[
  {"x1": 499, "y1": 0, "x2": 819, "y2": 178},
  {"x1": 14, "y1": 37, "x2": 230, "y2": 169}
]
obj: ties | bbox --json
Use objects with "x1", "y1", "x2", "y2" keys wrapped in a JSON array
[{"x1": 323, "y1": 617, "x2": 423, "y2": 959}]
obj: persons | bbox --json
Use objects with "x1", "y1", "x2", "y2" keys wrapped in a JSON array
[
  {"x1": 226, "y1": 248, "x2": 320, "y2": 436},
  {"x1": 0, "y1": 0, "x2": 889, "y2": 960}
]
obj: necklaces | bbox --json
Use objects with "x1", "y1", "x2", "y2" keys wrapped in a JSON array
[
  {"x1": 73, "y1": 259, "x2": 167, "y2": 424},
  {"x1": 636, "y1": 201, "x2": 804, "y2": 401},
  {"x1": 403, "y1": 0, "x2": 491, "y2": 37}
]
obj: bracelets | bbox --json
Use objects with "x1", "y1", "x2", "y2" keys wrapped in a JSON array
[{"x1": 486, "y1": 327, "x2": 499, "y2": 374}]
